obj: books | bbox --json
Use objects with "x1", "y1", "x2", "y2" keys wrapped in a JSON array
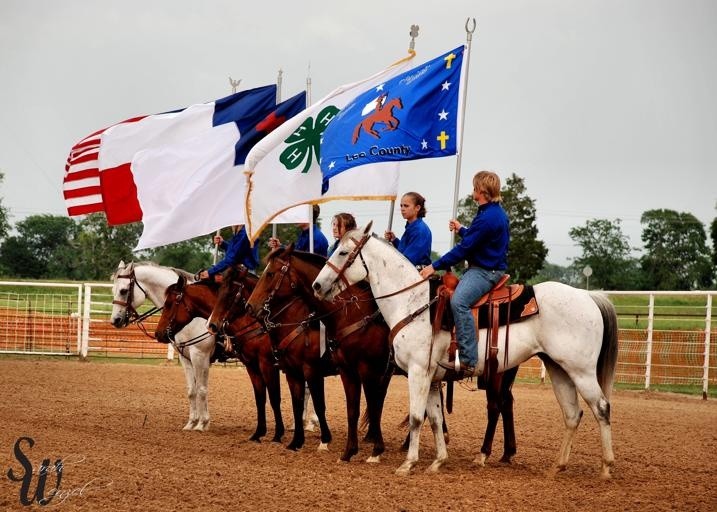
[
  {"x1": 62, "y1": 108, "x2": 194, "y2": 217},
  {"x1": 244, "y1": 49, "x2": 415, "y2": 248},
  {"x1": 319, "y1": 42, "x2": 467, "y2": 196},
  {"x1": 130, "y1": 87, "x2": 306, "y2": 252},
  {"x1": 99, "y1": 84, "x2": 276, "y2": 228}
]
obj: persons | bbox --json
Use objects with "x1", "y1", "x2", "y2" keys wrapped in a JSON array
[
  {"x1": 383, "y1": 192, "x2": 432, "y2": 274},
  {"x1": 268, "y1": 204, "x2": 329, "y2": 261},
  {"x1": 199, "y1": 225, "x2": 260, "y2": 278},
  {"x1": 420, "y1": 171, "x2": 510, "y2": 374},
  {"x1": 326, "y1": 213, "x2": 357, "y2": 258}
]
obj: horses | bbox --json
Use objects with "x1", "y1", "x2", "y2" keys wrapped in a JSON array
[
  {"x1": 312, "y1": 220, "x2": 619, "y2": 483},
  {"x1": 206, "y1": 262, "x2": 454, "y2": 450},
  {"x1": 110, "y1": 258, "x2": 320, "y2": 433},
  {"x1": 245, "y1": 240, "x2": 517, "y2": 467},
  {"x1": 155, "y1": 271, "x2": 370, "y2": 443}
]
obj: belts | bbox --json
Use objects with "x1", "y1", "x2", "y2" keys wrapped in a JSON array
[{"x1": 468, "y1": 264, "x2": 480, "y2": 269}]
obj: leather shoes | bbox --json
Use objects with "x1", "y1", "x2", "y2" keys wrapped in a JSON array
[{"x1": 437, "y1": 360, "x2": 475, "y2": 377}]
